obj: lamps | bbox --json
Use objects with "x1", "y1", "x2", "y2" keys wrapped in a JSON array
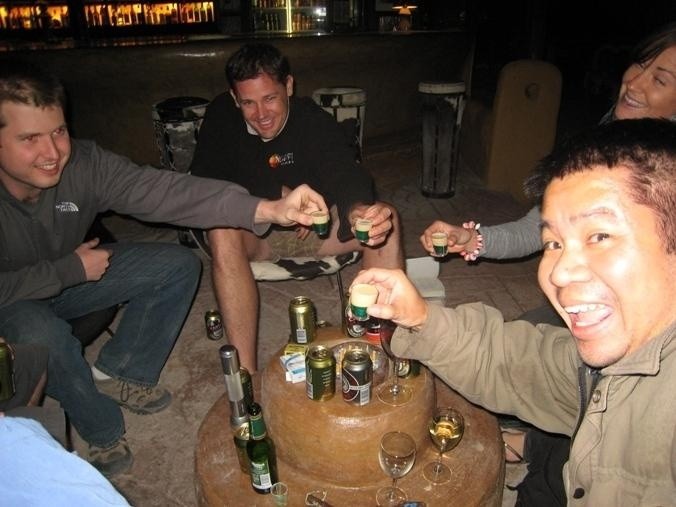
[{"x1": 391, "y1": 0, "x2": 418, "y2": 16}]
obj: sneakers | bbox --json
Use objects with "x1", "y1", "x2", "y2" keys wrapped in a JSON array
[
  {"x1": 86, "y1": 436, "x2": 134, "y2": 479},
  {"x1": 91, "y1": 364, "x2": 172, "y2": 415}
]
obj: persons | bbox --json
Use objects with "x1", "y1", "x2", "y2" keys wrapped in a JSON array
[
  {"x1": 0, "y1": 53, "x2": 330, "y2": 480},
  {"x1": 0, "y1": 410, "x2": 134, "y2": 507},
  {"x1": 339, "y1": 113, "x2": 676, "y2": 506},
  {"x1": 0, "y1": 334, "x2": 70, "y2": 453},
  {"x1": 417, "y1": 30, "x2": 676, "y2": 466},
  {"x1": 181, "y1": 40, "x2": 405, "y2": 386}
]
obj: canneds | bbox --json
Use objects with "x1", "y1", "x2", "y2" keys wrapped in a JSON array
[
  {"x1": 342, "y1": 291, "x2": 367, "y2": 338},
  {"x1": 397, "y1": 360, "x2": 420, "y2": 380},
  {"x1": 342, "y1": 349, "x2": 374, "y2": 406},
  {"x1": 233, "y1": 421, "x2": 252, "y2": 475},
  {"x1": 205, "y1": 310, "x2": 223, "y2": 340},
  {"x1": 289, "y1": 295, "x2": 316, "y2": 344},
  {"x1": 239, "y1": 367, "x2": 254, "y2": 408},
  {"x1": 305, "y1": 344, "x2": 336, "y2": 402}
]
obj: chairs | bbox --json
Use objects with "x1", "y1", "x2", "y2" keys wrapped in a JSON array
[
  {"x1": 64, "y1": 218, "x2": 131, "y2": 452},
  {"x1": 188, "y1": 228, "x2": 363, "y2": 338}
]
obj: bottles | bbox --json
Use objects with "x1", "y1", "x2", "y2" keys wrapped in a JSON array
[
  {"x1": 218, "y1": 344, "x2": 252, "y2": 473},
  {"x1": 0, "y1": 0, "x2": 216, "y2": 27},
  {"x1": 242, "y1": 402, "x2": 279, "y2": 494},
  {"x1": 252, "y1": 0, "x2": 333, "y2": 36}
]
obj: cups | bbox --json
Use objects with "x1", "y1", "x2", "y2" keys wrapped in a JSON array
[
  {"x1": 354, "y1": 219, "x2": 372, "y2": 243},
  {"x1": 350, "y1": 283, "x2": 379, "y2": 321},
  {"x1": 270, "y1": 482, "x2": 289, "y2": 506},
  {"x1": 431, "y1": 232, "x2": 449, "y2": 256},
  {"x1": 310, "y1": 210, "x2": 330, "y2": 236}
]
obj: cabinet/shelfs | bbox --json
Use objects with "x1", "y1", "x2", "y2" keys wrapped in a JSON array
[{"x1": 0, "y1": 0, "x2": 363, "y2": 34}]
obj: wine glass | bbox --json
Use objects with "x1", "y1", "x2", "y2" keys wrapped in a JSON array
[
  {"x1": 422, "y1": 407, "x2": 465, "y2": 486},
  {"x1": 374, "y1": 430, "x2": 415, "y2": 506},
  {"x1": 376, "y1": 318, "x2": 414, "y2": 407}
]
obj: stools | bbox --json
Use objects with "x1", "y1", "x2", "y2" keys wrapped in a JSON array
[
  {"x1": 311, "y1": 87, "x2": 369, "y2": 161},
  {"x1": 416, "y1": 78, "x2": 467, "y2": 199},
  {"x1": 151, "y1": 96, "x2": 212, "y2": 174}
]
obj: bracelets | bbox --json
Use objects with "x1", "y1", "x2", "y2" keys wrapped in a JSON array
[{"x1": 457, "y1": 219, "x2": 485, "y2": 263}]
